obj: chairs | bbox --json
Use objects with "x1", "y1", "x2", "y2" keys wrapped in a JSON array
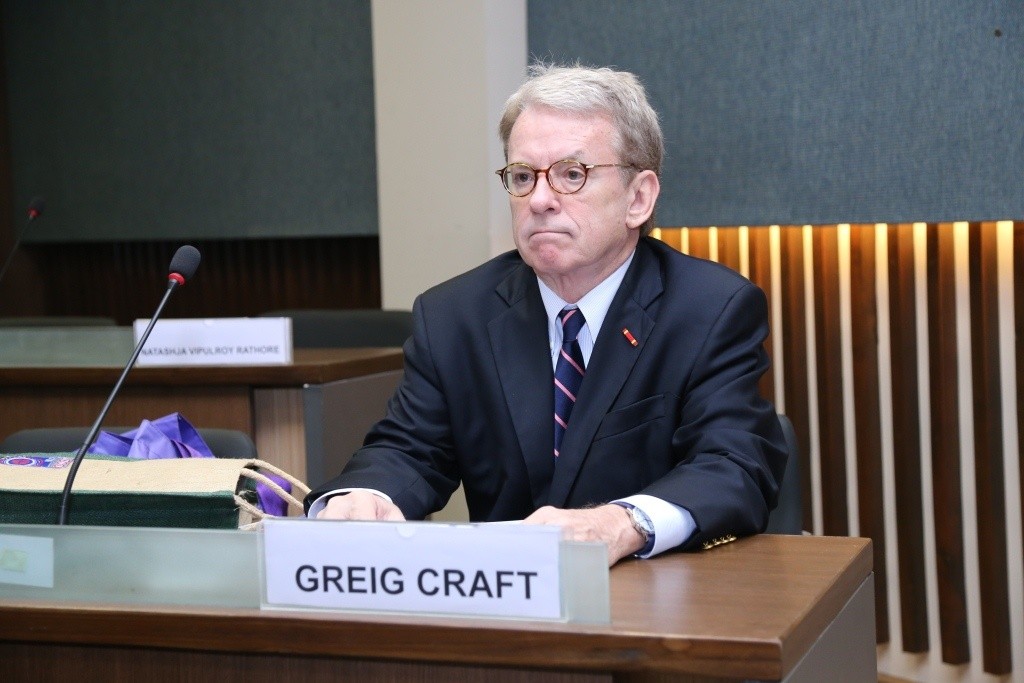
[{"x1": 264, "y1": 311, "x2": 413, "y2": 347}]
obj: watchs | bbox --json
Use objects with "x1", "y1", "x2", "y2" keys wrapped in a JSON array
[{"x1": 609, "y1": 502, "x2": 655, "y2": 557}]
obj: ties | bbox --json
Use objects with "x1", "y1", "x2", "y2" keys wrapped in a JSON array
[{"x1": 552, "y1": 308, "x2": 586, "y2": 470}]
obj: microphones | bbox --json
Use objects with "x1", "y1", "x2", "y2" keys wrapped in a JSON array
[{"x1": 59, "y1": 244, "x2": 201, "y2": 524}]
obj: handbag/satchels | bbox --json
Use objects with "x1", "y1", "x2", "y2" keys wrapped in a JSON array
[{"x1": 0, "y1": 411, "x2": 312, "y2": 531}]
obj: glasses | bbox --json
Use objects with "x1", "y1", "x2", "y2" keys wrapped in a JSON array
[{"x1": 495, "y1": 158, "x2": 643, "y2": 197}]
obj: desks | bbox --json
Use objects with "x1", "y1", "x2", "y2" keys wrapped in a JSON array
[
  {"x1": 1, "y1": 346, "x2": 403, "y2": 518},
  {"x1": 0, "y1": 535, "x2": 877, "y2": 683}
]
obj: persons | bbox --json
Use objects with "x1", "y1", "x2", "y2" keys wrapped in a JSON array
[{"x1": 303, "y1": 68, "x2": 791, "y2": 570}]
